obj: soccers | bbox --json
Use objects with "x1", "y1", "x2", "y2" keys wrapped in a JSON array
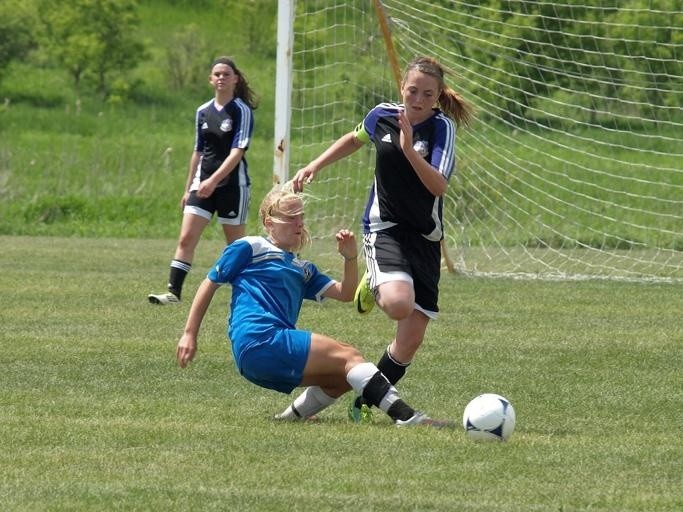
[{"x1": 461, "y1": 393, "x2": 515, "y2": 442}]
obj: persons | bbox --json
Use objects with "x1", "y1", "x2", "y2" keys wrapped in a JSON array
[
  {"x1": 289, "y1": 56, "x2": 481, "y2": 427},
  {"x1": 173, "y1": 179, "x2": 458, "y2": 433},
  {"x1": 145, "y1": 57, "x2": 262, "y2": 304}
]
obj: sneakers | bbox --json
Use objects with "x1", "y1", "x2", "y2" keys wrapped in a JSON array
[
  {"x1": 148, "y1": 291, "x2": 178, "y2": 306},
  {"x1": 354, "y1": 269, "x2": 375, "y2": 316},
  {"x1": 347, "y1": 399, "x2": 374, "y2": 423},
  {"x1": 394, "y1": 411, "x2": 448, "y2": 428}
]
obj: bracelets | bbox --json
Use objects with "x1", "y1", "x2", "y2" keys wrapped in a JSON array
[{"x1": 345, "y1": 256, "x2": 357, "y2": 261}]
